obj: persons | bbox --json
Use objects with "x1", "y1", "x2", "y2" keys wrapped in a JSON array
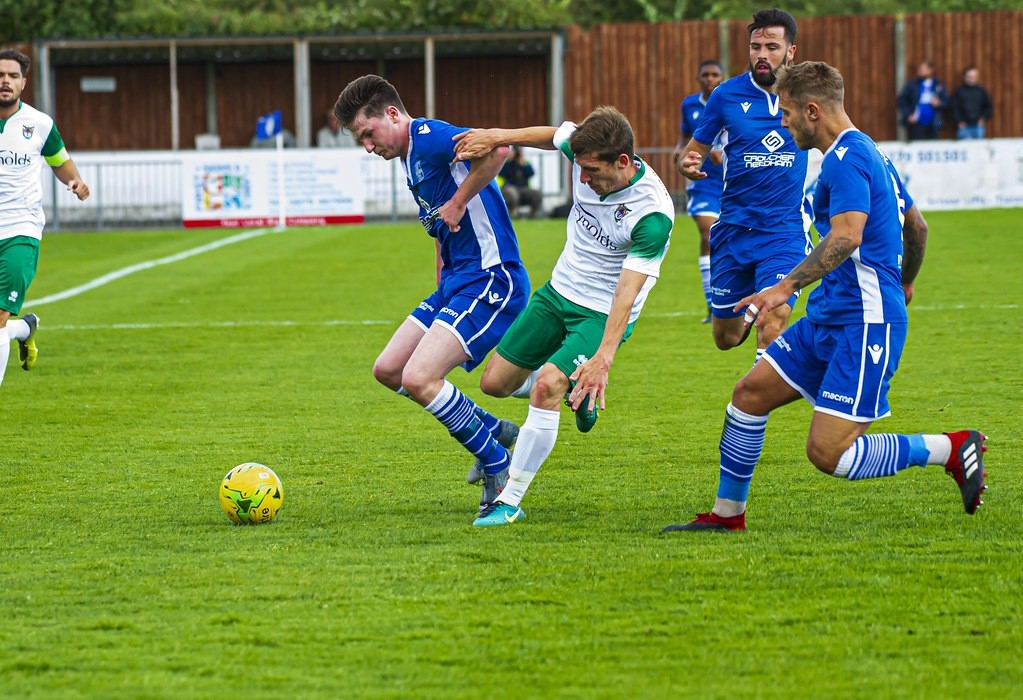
[
  {"x1": 316, "y1": 107, "x2": 361, "y2": 148},
  {"x1": 678, "y1": 9, "x2": 825, "y2": 371},
  {"x1": 334, "y1": 74, "x2": 531, "y2": 516},
  {"x1": 249, "y1": 113, "x2": 296, "y2": 149},
  {"x1": 659, "y1": 62, "x2": 990, "y2": 533},
  {"x1": 948, "y1": 65, "x2": 992, "y2": 141},
  {"x1": 0, "y1": 48, "x2": 88, "y2": 388},
  {"x1": 498, "y1": 143, "x2": 543, "y2": 218},
  {"x1": 896, "y1": 59, "x2": 947, "y2": 140},
  {"x1": 452, "y1": 104, "x2": 675, "y2": 526},
  {"x1": 674, "y1": 54, "x2": 729, "y2": 327}
]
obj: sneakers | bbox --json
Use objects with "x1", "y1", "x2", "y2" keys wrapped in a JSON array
[
  {"x1": 472, "y1": 496, "x2": 528, "y2": 528},
  {"x1": 466, "y1": 421, "x2": 519, "y2": 486},
  {"x1": 658, "y1": 512, "x2": 746, "y2": 533},
  {"x1": 19, "y1": 314, "x2": 39, "y2": 371},
  {"x1": 478, "y1": 448, "x2": 512, "y2": 510},
  {"x1": 565, "y1": 381, "x2": 599, "y2": 434},
  {"x1": 939, "y1": 430, "x2": 990, "y2": 515}
]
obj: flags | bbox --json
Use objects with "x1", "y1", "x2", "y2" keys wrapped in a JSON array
[{"x1": 255, "y1": 110, "x2": 282, "y2": 139}]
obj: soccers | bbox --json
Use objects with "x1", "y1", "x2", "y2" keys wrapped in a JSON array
[{"x1": 218, "y1": 461, "x2": 284, "y2": 524}]
